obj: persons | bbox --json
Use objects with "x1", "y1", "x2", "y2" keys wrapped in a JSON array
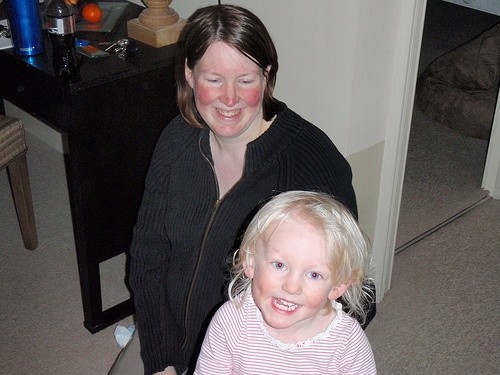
[
  {"x1": 110, "y1": 5, "x2": 377, "y2": 375},
  {"x1": 193, "y1": 190, "x2": 377, "y2": 375}
]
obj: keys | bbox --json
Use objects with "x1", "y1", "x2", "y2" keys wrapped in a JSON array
[{"x1": 104, "y1": 36, "x2": 129, "y2": 60}]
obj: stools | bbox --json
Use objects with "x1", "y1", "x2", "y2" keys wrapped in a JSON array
[{"x1": 0, "y1": 115, "x2": 39, "y2": 251}]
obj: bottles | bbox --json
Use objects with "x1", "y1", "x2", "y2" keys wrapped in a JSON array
[{"x1": 46, "y1": 0, "x2": 81, "y2": 77}]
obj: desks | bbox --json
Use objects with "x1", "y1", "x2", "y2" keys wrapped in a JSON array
[{"x1": 0, "y1": 0, "x2": 181, "y2": 334}]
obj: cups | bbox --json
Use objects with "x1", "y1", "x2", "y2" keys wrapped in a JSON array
[{"x1": 6, "y1": 0, "x2": 44, "y2": 56}]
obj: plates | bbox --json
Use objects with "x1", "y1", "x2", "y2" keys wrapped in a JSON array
[{"x1": 43, "y1": 2, "x2": 129, "y2": 32}]
